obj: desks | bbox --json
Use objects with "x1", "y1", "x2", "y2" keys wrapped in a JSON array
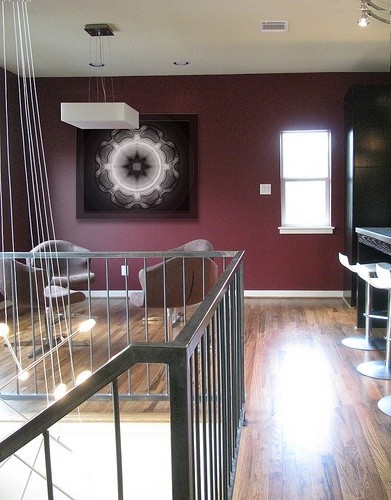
[{"x1": 355, "y1": 227, "x2": 391, "y2": 328}]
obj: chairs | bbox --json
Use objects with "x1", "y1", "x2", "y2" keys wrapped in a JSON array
[
  {"x1": 338, "y1": 252, "x2": 391, "y2": 417},
  {"x1": 25, "y1": 240, "x2": 95, "y2": 326},
  {"x1": 130, "y1": 239, "x2": 218, "y2": 329},
  {"x1": 0, "y1": 258, "x2": 90, "y2": 358}
]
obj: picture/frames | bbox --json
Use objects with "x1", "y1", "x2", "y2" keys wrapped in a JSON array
[{"x1": 75, "y1": 114, "x2": 199, "y2": 219}]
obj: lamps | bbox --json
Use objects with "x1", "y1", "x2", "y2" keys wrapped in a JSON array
[
  {"x1": 60, "y1": 24, "x2": 140, "y2": 129},
  {"x1": 358, "y1": 0, "x2": 391, "y2": 26}
]
